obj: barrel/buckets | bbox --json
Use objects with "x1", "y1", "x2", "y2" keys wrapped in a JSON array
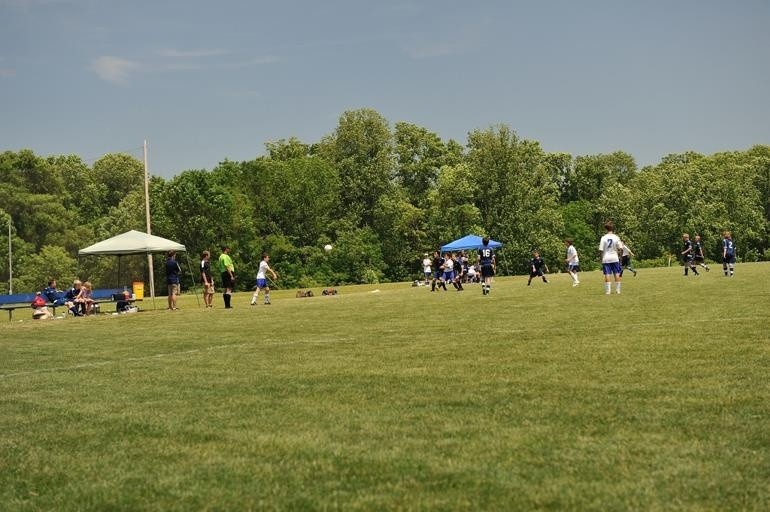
[{"x1": 133, "y1": 281, "x2": 143, "y2": 299}]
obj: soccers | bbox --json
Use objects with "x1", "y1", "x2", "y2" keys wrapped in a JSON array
[{"x1": 324, "y1": 244, "x2": 333, "y2": 252}]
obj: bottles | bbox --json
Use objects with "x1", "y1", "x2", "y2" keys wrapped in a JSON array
[
  {"x1": 51, "y1": 311, "x2": 68, "y2": 320},
  {"x1": 122, "y1": 286, "x2": 129, "y2": 300}
]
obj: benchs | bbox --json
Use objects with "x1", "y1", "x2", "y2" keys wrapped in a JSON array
[{"x1": 1, "y1": 297, "x2": 146, "y2": 320}]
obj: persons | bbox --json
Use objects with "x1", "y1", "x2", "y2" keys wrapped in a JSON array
[
  {"x1": 565, "y1": 240, "x2": 580, "y2": 287},
  {"x1": 217, "y1": 246, "x2": 235, "y2": 309},
  {"x1": 77, "y1": 281, "x2": 96, "y2": 315},
  {"x1": 62, "y1": 280, "x2": 86, "y2": 315},
  {"x1": 597, "y1": 224, "x2": 624, "y2": 295},
  {"x1": 36, "y1": 279, "x2": 83, "y2": 316},
  {"x1": 692, "y1": 235, "x2": 710, "y2": 275},
  {"x1": 680, "y1": 233, "x2": 698, "y2": 276},
  {"x1": 618, "y1": 239, "x2": 637, "y2": 279},
  {"x1": 527, "y1": 252, "x2": 551, "y2": 288},
  {"x1": 200, "y1": 250, "x2": 214, "y2": 308},
  {"x1": 250, "y1": 252, "x2": 277, "y2": 305},
  {"x1": 420, "y1": 248, "x2": 479, "y2": 292},
  {"x1": 477, "y1": 237, "x2": 496, "y2": 295},
  {"x1": 721, "y1": 231, "x2": 736, "y2": 278},
  {"x1": 165, "y1": 251, "x2": 179, "y2": 310}
]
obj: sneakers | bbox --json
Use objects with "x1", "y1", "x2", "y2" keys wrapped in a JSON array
[
  {"x1": 225, "y1": 306, "x2": 233, "y2": 309},
  {"x1": 527, "y1": 281, "x2": 531, "y2": 285},
  {"x1": 425, "y1": 281, "x2": 483, "y2": 284},
  {"x1": 605, "y1": 289, "x2": 612, "y2": 295},
  {"x1": 615, "y1": 289, "x2": 621, "y2": 294},
  {"x1": 543, "y1": 279, "x2": 547, "y2": 282},
  {"x1": 683, "y1": 274, "x2": 688, "y2": 276},
  {"x1": 572, "y1": 281, "x2": 580, "y2": 287},
  {"x1": 206, "y1": 305, "x2": 214, "y2": 308},
  {"x1": 250, "y1": 302, "x2": 258, "y2": 305},
  {"x1": 167, "y1": 306, "x2": 180, "y2": 311},
  {"x1": 483, "y1": 292, "x2": 489, "y2": 295},
  {"x1": 724, "y1": 274, "x2": 734, "y2": 278},
  {"x1": 74, "y1": 313, "x2": 93, "y2": 316},
  {"x1": 706, "y1": 267, "x2": 710, "y2": 272},
  {"x1": 695, "y1": 273, "x2": 699, "y2": 276},
  {"x1": 264, "y1": 302, "x2": 271, "y2": 305},
  {"x1": 431, "y1": 286, "x2": 464, "y2": 291},
  {"x1": 633, "y1": 271, "x2": 637, "y2": 277}
]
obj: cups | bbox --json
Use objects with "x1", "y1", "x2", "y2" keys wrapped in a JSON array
[{"x1": 131, "y1": 294, "x2": 137, "y2": 300}]
